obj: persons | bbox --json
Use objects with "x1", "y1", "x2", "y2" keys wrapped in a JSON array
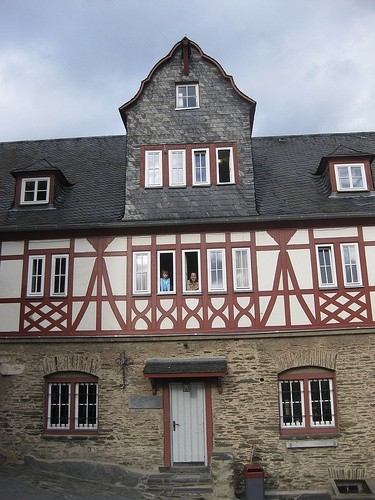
[
  {"x1": 186, "y1": 272, "x2": 199, "y2": 290},
  {"x1": 160, "y1": 270, "x2": 171, "y2": 291}
]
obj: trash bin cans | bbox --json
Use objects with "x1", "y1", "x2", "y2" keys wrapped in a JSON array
[{"x1": 243, "y1": 464, "x2": 265, "y2": 500}]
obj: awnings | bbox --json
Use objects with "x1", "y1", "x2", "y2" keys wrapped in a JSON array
[{"x1": 143, "y1": 356, "x2": 228, "y2": 395}]
